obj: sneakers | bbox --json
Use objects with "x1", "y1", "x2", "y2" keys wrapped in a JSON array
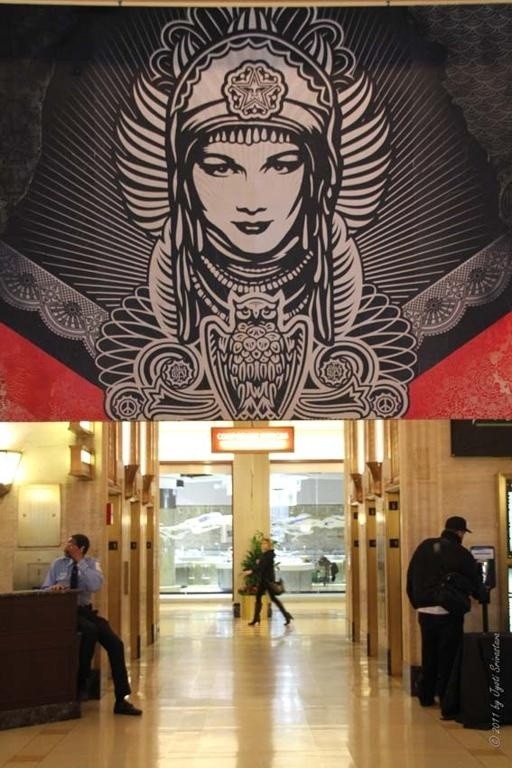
[
  {"x1": 417, "y1": 694, "x2": 448, "y2": 707},
  {"x1": 112, "y1": 698, "x2": 145, "y2": 717}
]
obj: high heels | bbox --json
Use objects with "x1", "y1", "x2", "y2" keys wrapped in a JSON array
[
  {"x1": 283, "y1": 611, "x2": 294, "y2": 626},
  {"x1": 246, "y1": 615, "x2": 260, "y2": 626}
]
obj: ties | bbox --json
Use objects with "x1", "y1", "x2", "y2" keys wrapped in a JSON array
[{"x1": 70, "y1": 561, "x2": 79, "y2": 591}]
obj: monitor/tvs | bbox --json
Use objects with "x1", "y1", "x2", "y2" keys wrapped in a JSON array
[{"x1": 450, "y1": 419, "x2": 512, "y2": 458}]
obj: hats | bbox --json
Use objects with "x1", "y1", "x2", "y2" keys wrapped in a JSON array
[{"x1": 443, "y1": 515, "x2": 474, "y2": 535}]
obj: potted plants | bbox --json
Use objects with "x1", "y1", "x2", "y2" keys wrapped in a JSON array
[{"x1": 237, "y1": 532, "x2": 279, "y2": 622}]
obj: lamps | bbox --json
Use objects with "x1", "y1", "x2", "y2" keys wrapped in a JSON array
[{"x1": 1, "y1": 450, "x2": 25, "y2": 498}]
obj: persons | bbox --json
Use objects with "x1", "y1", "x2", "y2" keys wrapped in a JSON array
[
  {"x1": 146, "y1": 126, "x2": 364, "y2": 344},
  {"x1": 41, "y1": 533, "x2": 143, "y2": 717},
  {"x1": 246, "y1": 539, "x2": 295, "y2": 627},
  {"x1": 404, "y1": 515, "x2": 488, "y2": 722}
]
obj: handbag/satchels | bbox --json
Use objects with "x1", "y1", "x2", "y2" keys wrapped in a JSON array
[
  {"x1": 435, "y1": 571, "x2": 472, "y2": 617},
  {"x1": 267, "y1": 578, "x2": 285, "y2": 595}
]
